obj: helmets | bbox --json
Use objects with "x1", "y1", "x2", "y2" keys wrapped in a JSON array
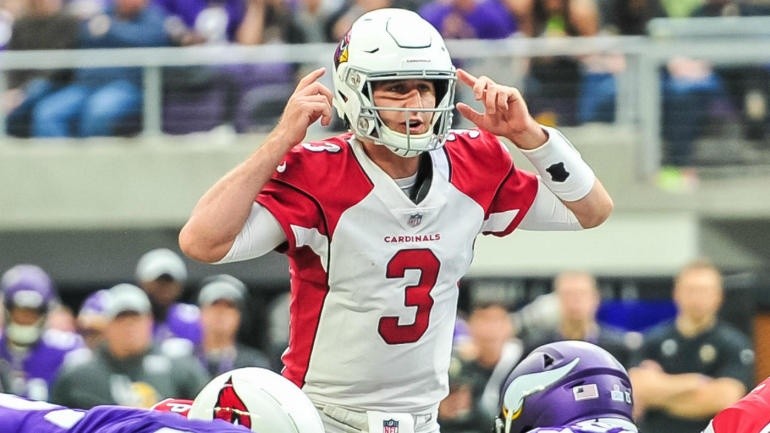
[
  {"x1": 0, "y1": 263, "x2": 55, "y2": 309},
  {"x1": 333, "y1": 8, "x2": 458, "y2": 158},
  {"x1": 492, "y1": 340, "x2": 639, "y2": 433},
  {"x1": 75, "y1": 289, "x2": 111, "y2": 333},
  {"x1": 187, "y1": 365, "x2": 326, "y2": 433}
]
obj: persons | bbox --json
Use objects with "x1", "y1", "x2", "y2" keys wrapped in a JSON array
[
  {"x1": 0, "y1": 248, "x2": 293, "y2": 433},
  {"x1": 434, "y1": 303, "x2": 524, "y2": 433},
  {"x1": 151, "y1": 367, "x2": 323, "y2": 433},
  {"x1": 180, "y1": 6, "x2": 614, "y2": 433},
  {"x1": 701, "y1": 375, "x2": 770, "y2": 433},
  {"x1": 626, "y1": 263, "x2": 755, "y2": 433},
  {"x1": 522, "y1": 267, "x2": 633, "y2": 369},
  {"x1": 491, "y1": 340, "x2": 641, "y2": 433},
  {"x1": 0, "y1": 0, "x2": 770, "y2": 173}
]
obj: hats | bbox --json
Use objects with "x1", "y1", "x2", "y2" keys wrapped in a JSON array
[
  {"x1": 198, "y1": 273, "x2": 250, "y2": 310},
  {"x1": 139, "y1": 247, "x2": 189, "y2": 287},
  {"x1": 103, "y1": 285, "x2": 150, "y2": 320}
]
